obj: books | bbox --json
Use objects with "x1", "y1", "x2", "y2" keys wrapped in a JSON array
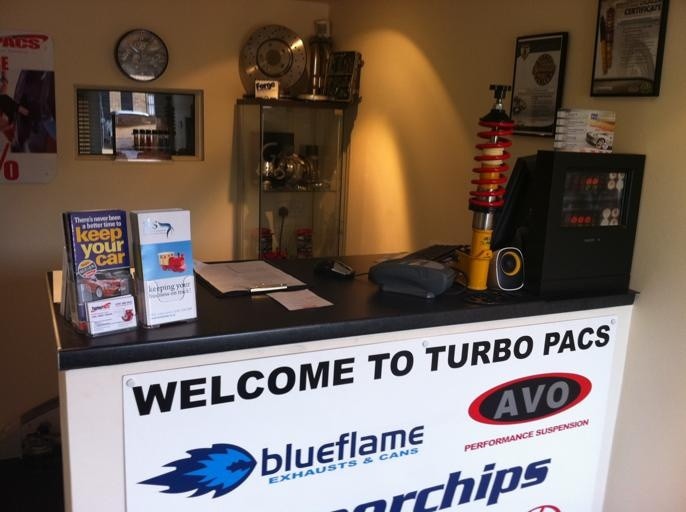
[
  {"x1": 128, "y1": 208, "x2": 198, "y2": 325},
  {"x1": 59, "y1": 207, "x2": 131, "y2": 333}
]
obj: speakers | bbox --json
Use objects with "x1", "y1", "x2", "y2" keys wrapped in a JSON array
[{"x1": 487, "y1": 246, "x2": 527, "y2": 301}]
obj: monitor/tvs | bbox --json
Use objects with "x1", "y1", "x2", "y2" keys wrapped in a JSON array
[{"x1": 491, "y1": 154, "x2": 537, "y2": 250}]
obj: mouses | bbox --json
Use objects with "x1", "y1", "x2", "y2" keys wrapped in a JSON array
[{"x1": 313, "y1": 258, "x2": 355, "y2": 280}]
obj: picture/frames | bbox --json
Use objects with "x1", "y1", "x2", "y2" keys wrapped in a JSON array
[
  {"x1": 509, "y1": 31, "x2": 568, "y2": 139},
  {"x1": 589, "y1": 0, "x2": 670, "y2": 96}
]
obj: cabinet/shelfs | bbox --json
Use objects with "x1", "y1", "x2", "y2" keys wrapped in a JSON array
[{"x1": 237, "y1": 97, "x2": 350, "y2": 261}]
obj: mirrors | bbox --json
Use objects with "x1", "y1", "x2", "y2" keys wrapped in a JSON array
[{"x1": 73, "y1": 83, "x2": 205, "y2": 161}]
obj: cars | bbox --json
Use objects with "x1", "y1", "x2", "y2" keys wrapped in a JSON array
[{"x1": 84, "y1": 270, "x2": 127, "y2": 299}]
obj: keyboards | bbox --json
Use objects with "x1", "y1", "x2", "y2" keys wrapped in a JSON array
[{"x1": 402, "y1": 244, "x2": 462, "y2": 263}]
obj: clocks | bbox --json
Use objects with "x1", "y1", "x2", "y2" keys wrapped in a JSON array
[{"x1": 114, "y1": 29, "x2": 169, "y2": 83}]
